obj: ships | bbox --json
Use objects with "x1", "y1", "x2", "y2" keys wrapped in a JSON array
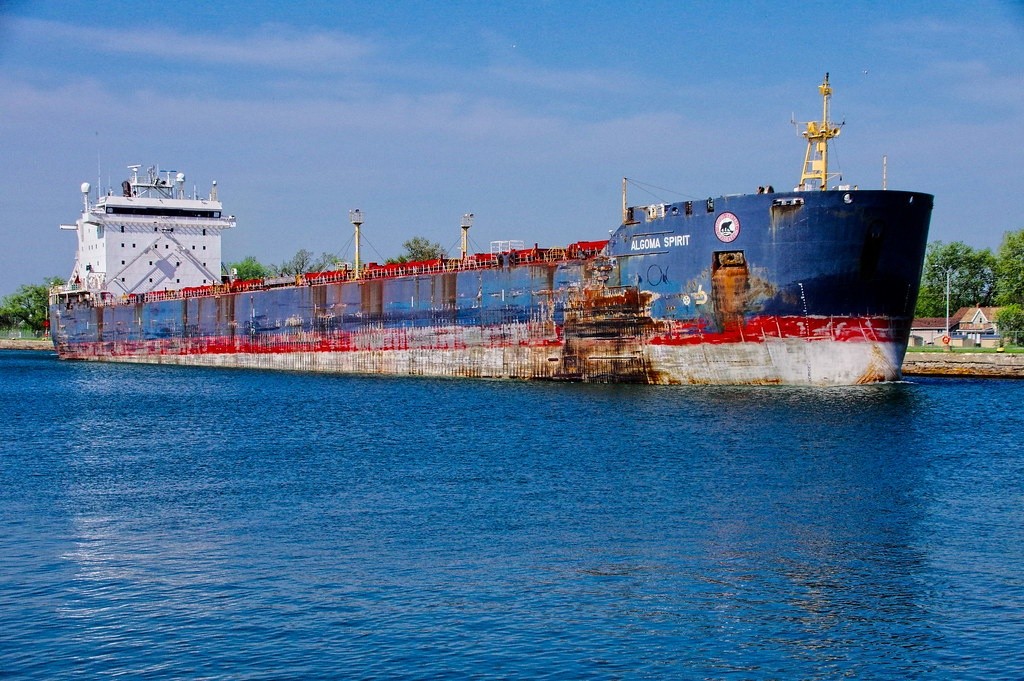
[{"x1": 47, "y1": 130, "x2": 935, "y2": 388}]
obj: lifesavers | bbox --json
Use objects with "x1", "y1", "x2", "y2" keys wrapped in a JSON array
[{"x1": 942, "y1": 336, "x2": 950, "y2": 344}]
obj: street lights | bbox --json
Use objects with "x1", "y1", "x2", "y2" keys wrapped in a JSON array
[{"x1": 931, "y1": 264, "x2": 950, "y2": 338}]
